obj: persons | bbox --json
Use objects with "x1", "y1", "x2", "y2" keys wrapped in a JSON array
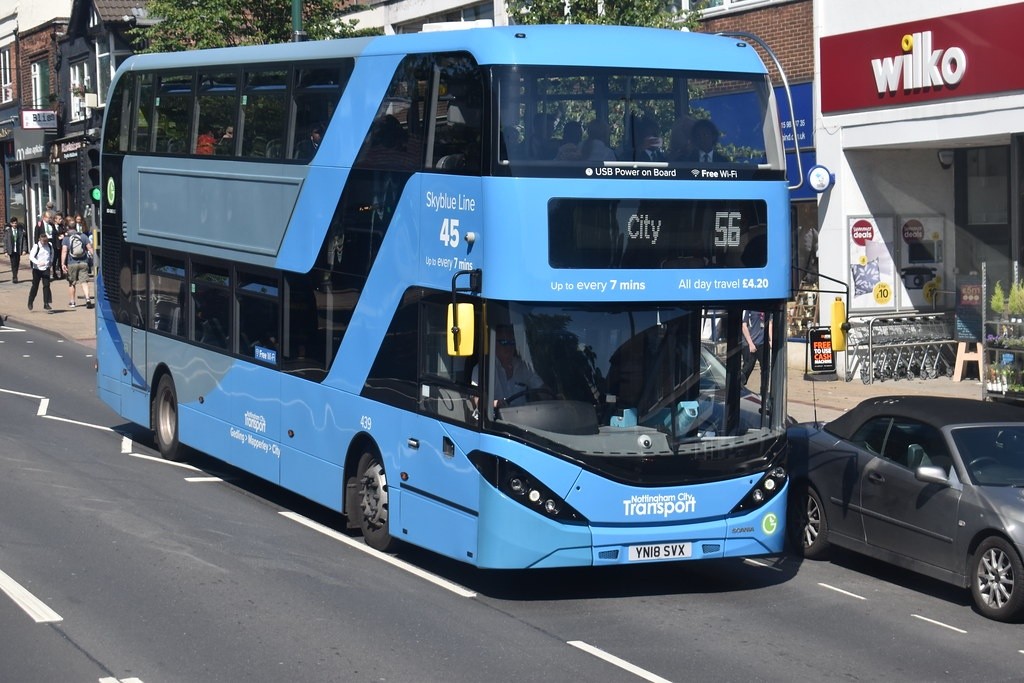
[
  {"x1": 741, "y1": 310, "x2": 773, "y2": 395},
  {"x1": 28, "y1": 233, "x2": 54, "y2": 310},
  {"x1": 472, "y1": 324, "x2": 552, "y2": 418},
  {"x1": 3, "y1": 216, "x2": 28, "y2": 283},
  {"x1": 298, "y1": 112, "x2": 730, "y2": 169},
  {"x1": 34, "y1": 202, "x2": 89, "y2": 281},
  {"x1": 61, "y1": 220, "x2": 93, "y2": 310}
]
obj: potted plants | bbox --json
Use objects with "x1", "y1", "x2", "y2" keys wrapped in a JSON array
[{"x1": 985, "y1": 283, "x2": 1024, "y2": 398}]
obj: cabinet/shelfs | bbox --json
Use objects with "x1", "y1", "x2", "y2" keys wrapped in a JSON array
[{"x1": 980, "y1": 263, "x2": 1023, "y2": 404}]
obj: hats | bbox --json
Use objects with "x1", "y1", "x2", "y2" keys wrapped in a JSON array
[{"x1": 47, "y1": 202, "x2": 53, "y2": 208}]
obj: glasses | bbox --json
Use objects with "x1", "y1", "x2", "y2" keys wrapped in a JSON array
[{"x1": 497, "y1": 338, "x2": 516, "y2": 346}]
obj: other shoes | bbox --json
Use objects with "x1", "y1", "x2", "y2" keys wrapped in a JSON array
[
  {"x1": 85, "y1": 298, "x2": 92, "y2": 309},
  {"x1": 13, "y1": 277, "x2": 18, "y2": 283},
  {"x1": 53, "y1": 273, "x2": 58, "y2": 280},
  {"x1": 28, "y1": 302, "x2": 33, "y2": 310},
  {"x1": 69, "y1": 302, "x2": 75, "y2": 308},
  {"x1": 44, "y1": 304, "x2": 51, "y2": 309},
  {"x1": 61, "y1": 273, "x2": 66, "y2": 279}
]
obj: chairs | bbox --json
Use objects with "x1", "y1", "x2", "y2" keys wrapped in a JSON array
[
  {"x1": 136, "y1": 125, "x2": 486, "y2": 175},
  {"x1": 122, "y1": 294, "x2": 342, "y2": 382}
]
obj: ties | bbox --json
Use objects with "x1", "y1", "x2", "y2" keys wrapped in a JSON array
[
  {"x1": 13, "y1": 230, "x2": 18, "y2": 242},
  {"x1": 703, "y1": 155, "x2": 709, "y2": 163},
  {"x1": 46, "y1": 224, "x2": 49, "y2": 234},
  {"x1": 78, "y1": 224, "x2": 81, "y2": 233},
  {"x1": 651, "y1": 153, "x2": 656, "y2": 162}
]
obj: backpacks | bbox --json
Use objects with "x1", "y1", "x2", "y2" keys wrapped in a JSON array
[
  {"x1": 30, "y1": 243, "x2": 51, "y2": 268},
  {"x1": 66, "y1": 233, "x2": 86, "y2": 258}
]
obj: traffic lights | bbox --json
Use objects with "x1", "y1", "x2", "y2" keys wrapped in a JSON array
[{"x1": 87, "y1": 146, "x2": 101, "y2": 204}]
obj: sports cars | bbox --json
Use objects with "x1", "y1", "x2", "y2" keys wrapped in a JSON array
[{"x1": 785, "y1": 394, "x2": 1024, "y2": 625}]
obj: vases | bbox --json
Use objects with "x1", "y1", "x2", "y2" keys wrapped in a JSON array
[
  {"x1": 986, "y1": 341, "x2": 994, "y2": 347},
  {"x1": 993, "y1": 342, "x2": 1001, "y2": 348}
]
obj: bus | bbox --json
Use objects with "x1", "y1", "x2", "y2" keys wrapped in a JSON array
[{"x1": 94, "y1": 22, "x2": 853, "y2": 572}]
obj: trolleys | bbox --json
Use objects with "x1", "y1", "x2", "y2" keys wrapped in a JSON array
[{"x1": 843, "y1": 310, "x2": 958, "y2": 385}]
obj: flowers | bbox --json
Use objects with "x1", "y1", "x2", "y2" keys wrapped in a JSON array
[{"x1": 987, "y1": 334, "x2": 1003, "y2": 343}]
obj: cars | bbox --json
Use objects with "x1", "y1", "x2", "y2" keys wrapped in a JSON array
[{"x1": 696, "y1": 343, "x2": 798, "y2": 442}]
